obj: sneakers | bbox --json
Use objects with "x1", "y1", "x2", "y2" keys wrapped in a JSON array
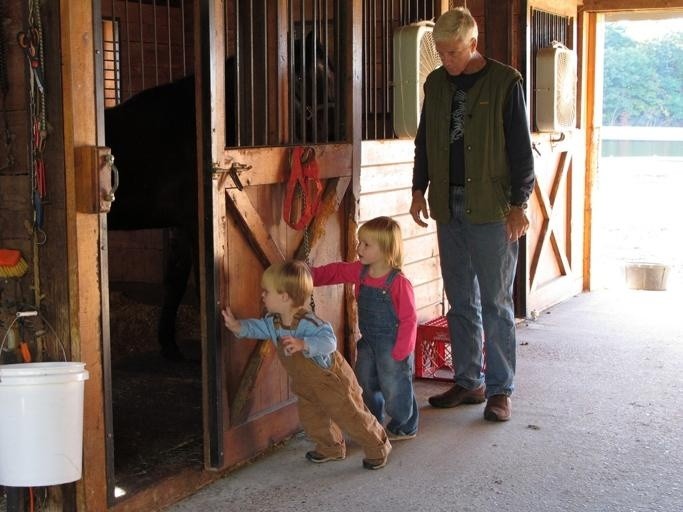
[
  {"x1": 383, "y1": 427, "x2": 417, "y2": 440},
  {"x1": 305, "y1": 451, "x2": 345, "y2": 463},
  {"x1": 362, "y1": 443, "x2": 392, "y2": 469}
]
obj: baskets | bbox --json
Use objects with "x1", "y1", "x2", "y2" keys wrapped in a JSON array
[{"x1": 414, "y1": 315, "x2": 487, "y2": 383}]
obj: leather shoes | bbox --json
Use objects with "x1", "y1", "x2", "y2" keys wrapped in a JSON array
[
  {"x1": 484, "y1": 394, "x2": 511, "y2": 421},
  {"x1": 428, "y1": 384, "x2": 485, "y2": 407}
]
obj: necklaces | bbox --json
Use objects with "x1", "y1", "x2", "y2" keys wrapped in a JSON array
[{"x1": 278, "y1": 319, "x2": 292, "y2": 330}]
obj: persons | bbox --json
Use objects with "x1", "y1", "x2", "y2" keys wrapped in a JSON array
[
  {"x1": 410, "y1": 7, "x2": 535, "y2": 421},
  {"x1": 310, "y1": 216, "x2": 419, "y2": 441},
  {"x1": 221, "y1": 260, "x2": 393, "y2": 470}
]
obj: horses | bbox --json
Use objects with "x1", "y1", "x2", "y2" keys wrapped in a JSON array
[{"x1": 104, "y1": 30, "x2": 334, "y2": 362}]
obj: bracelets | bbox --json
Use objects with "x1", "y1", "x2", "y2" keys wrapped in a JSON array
[{"x1": 510, "y1": 203, "x2": 528, "y2": 210}]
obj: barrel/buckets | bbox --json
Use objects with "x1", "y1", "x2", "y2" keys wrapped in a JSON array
[{"x1": 0, "y1": 310, "x2": 89, "y2": 487}]
